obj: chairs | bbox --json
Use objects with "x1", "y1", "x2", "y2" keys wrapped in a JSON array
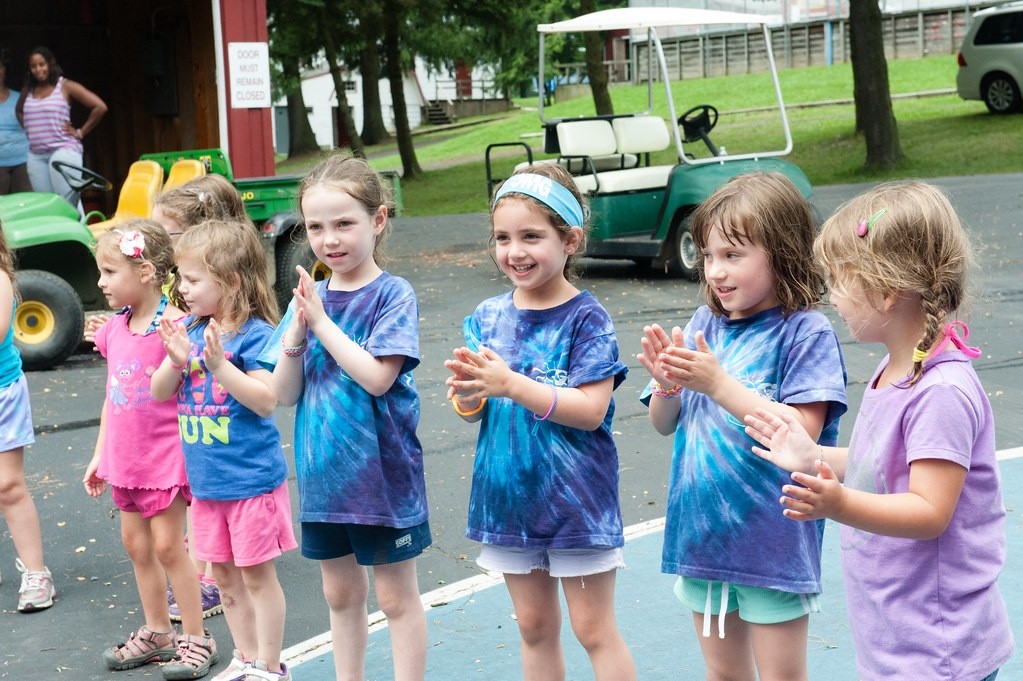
[
  {"x1": 87, "y1": 160, "x2": 163, "y2": 238},
  {"x1": 160, "y1": 160, "x2": 206, "y2": 199}
]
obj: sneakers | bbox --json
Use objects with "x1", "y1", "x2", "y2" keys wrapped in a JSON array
[
  {"x1": 210, "y1": 649, "x2": 292, "y2": 681},
  {"x1": 167, "y1": 573, "x2": 224, "y2": 620},
  {"x1": 18, "y1": 566, "x2": 56, "y2": 611}
]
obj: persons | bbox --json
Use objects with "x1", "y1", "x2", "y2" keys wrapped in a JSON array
[
  {"x1": 745, "y1": 179, "x2": 1013, "y2": 681},
  {"x1": 271, "y1": 151, "x2": 432, "y2": 681},
  {"x1": 81, "y1": 219, "x2": 222, "y2": 678},
  {"x1": 16, "y1": 46, "x2": 108, "y2": 225},
  {"x1": 0, "y1": 48, "x2": 33, "y2": 195},
  {"x1": 637, "y1": 171, "x2": 847, "y2": 681},
  {"x1": 150, "y1": 221, "x2": 296, "y2": 681},
  {"x1": 443, "y1": 164, "x2": 640, "y2": 681},
  {"x1": 0, "y1": 218, "x2": 58, "y2": 612},
  {"x1": 150, "y1": 174, "x2": 269, "y2": 623}
]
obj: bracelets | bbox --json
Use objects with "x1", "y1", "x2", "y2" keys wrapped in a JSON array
[
  {"x1": 650, "y1": 379, "x2": 682, "y2": 399},
  {"x1": 281, "y1": 332, "x2": 307, "y2": 356},
  {"x1": 450, "y1": 391, "x2": 488, "y2": 415},
  {"x1": 533, "y1": 385, "x2": 557, "y2": 421},
  {"x1": 170, "y1": 359, "x2": 188, "y2": 370},
  {"x1": 76, "y1": 128, "x2": 83, "y2": 139}
]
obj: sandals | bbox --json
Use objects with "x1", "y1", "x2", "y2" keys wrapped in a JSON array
[
  {"x1": 163, "y1": 629, "x2": 218, "y2": 678},
  {"x1": 102, "y1": 624, "x2": 180, "y2": 669}
]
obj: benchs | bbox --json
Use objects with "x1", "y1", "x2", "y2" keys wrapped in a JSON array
[{"x1": 516, "y1": 114, "x2": 676, "y2": 194}]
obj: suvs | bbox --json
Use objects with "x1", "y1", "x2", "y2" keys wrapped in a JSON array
[{"x1": 956, "y1": 1, "x2": 1023, "y2": 114}]
